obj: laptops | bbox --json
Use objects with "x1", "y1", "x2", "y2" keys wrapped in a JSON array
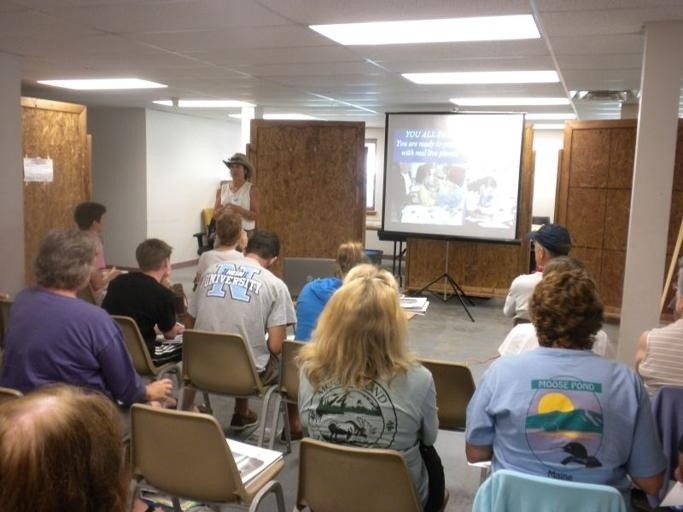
[{"x1": 284, "y1": 257, "x2": 337, "y2": 301}]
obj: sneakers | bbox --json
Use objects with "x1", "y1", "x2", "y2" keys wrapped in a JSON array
[
  {"x1": 278, "y1": 428, "x2": 303, "y2": 445},
  {"x1": 230, "y1": 409, "x2": 258, "y2": 430}
]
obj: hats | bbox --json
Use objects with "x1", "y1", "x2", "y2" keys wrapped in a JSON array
[
  {"x1": 525, "y1": 223, "x2": 569, "y2": 253},
  {"x1": 222, "y1": 153, "x2": 254, "y2": 176}
]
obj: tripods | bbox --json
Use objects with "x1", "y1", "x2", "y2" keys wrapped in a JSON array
[{"x1": 410, "y1": 240, "x2": 475, "y2": 322}]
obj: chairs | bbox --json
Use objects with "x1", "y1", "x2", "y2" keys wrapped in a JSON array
[
  {"x1": 0, "y1": 299, "x2": 682, "y2": 512},
  {"x1": 190, "y1": 224, "x2": 213, "y2": 292}
]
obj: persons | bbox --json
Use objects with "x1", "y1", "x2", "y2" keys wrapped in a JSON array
[
  {"x1": 389, "y1": 162, "x2": 518, "y2": 228},
  {"x1": 503, "y1": 224, "x2": 571, "y2": 327},
  {"x1": 294, "y1": 262, "x2": 449, "y2": 510},
  {"x1": 74, "y1": 202, "x2": 122, "y2": 306},
  {"x1": 192, "y1": 213, "x2": 248, "y2": 292},
  {"x1": 659, "y1": 433, "x2": 683, "y2": 510},
  {"x1": 293, "y1": 241, "x2": 372, "y2": 343},
  {"x1": 498, "y1": 256, "x2": 608, "y2": 357},
  {"x1": 634, "y1": 257, "x2": 683, "y2": 401},
  {"x1": 213, "y1": 154, "x2": 261, "y2": 252},
  {"x1": 183, "y1": 230, "x2": 311, "y2": 443},
  {"x1": 2, "y1": 383, "x2": 162, "y2": 510},
  {"x1": 1, "y1": 228, "x2": 178, "y2": 446},
  {"x1": 466, "y1": 269, "x2": 668, "y2": 510},
  {"x1": 101, "y1": 238, "x2": 206, "y2": 413}
]
